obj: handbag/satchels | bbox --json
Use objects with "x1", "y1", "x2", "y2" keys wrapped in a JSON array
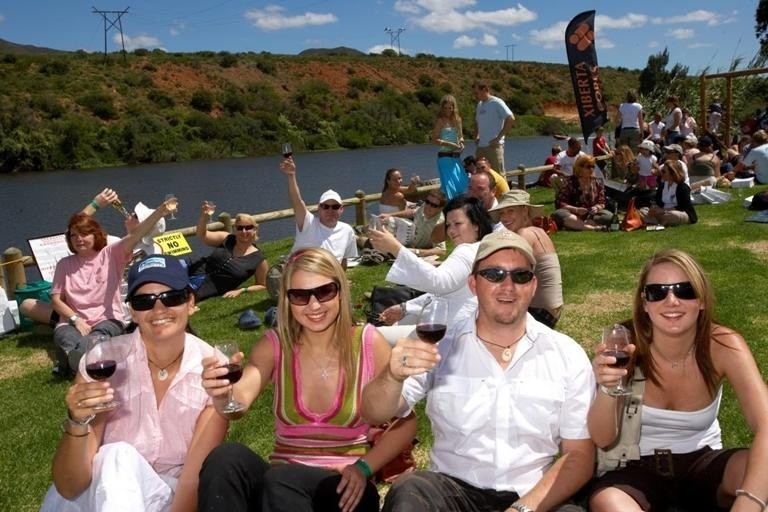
[
  {"x1": 367, "y1": 418, "x2": 420, "y2": 483},
  {"x1": 362, "y1": 286, "x2": 426, "y2": 327},
  {"x1": 623, "y1": 199, "x2": 646, "y2": 232}
]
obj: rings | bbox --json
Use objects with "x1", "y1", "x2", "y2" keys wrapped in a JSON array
[{"x1": 401, "y1": 353, "x2": 410, "y2": 366}]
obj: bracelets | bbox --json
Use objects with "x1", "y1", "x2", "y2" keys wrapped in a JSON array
[
  {"x1": 66, "y1": 407, "x2": 93, "y2": 427},
  {"x1": 735, "y1": 486, "x2": 768, "y2": 511},
  {"x1": 353, "y1": 457, "x2": 373, "y2": 479},
  {"x1": 507, "y1": 501, "x2": 531, "y2": 511},
  {"x1": 598, "y1": 376, "x2": 627, "y2": 395},
  {"x1": 60, "y1": 418, "x2": 94, "y2": 438}
]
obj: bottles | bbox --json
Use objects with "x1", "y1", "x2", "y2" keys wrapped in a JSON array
[
  {"x1": 111, "y1": 198, "x2": 131, "y2": 220},
  {"x1": 353, "y1": 304, "x2": 367, "y2": 326},
  {"x1": 609, "y1": 201, "x2": 619, "y2": 231}
]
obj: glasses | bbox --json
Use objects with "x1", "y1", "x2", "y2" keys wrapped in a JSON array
[
  {"x1": 236, "y1": 224, "x2": 254, "y2": 231},
  {"x1": 131, "y1": 288, "x2": 189, "y2": 311},
  {"x1": 474, "y1": 268, "x2": 535, "y2": 284},
  {"x1": 643, "y1": 281, "x2": 697, "y2": 303},
  {"x1": 426, "y1": 199, "x2": 440, "y2": 208},
  {"x1": 285, "y1": 282, "x2": 341, "y2": 306},
  {"x1": 321, "y1": 203, "x2": 342, "y2": 210},
  {"x1": 583, "y1": 163, "x2": 595, "y2": 171},
  {"x1": 134, "y1": 213, "x2": 138, "y2": 219}
]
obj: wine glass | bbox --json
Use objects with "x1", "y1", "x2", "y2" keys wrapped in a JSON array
[
  {"x1": 204, "y1": 200, "x2": 216, "y2": 225},
  {"x1": 280, "y1": 142, "x2": 296, "y2": 168},
  {"x1": 597, "y1": 324, "x2": 635, "y2": 398},
  {"x1": 416, "y1": 296, "x2": 450, "y2": 345},
  {"x1": 84, "y1": 331, "x2": 122, "y2": 412},
  {"x1": 212, "y1": 338, "x2": 249, "y2": 414},
  {"x1": 164, "y1": 193, "x2": 180, "y2": 220}
]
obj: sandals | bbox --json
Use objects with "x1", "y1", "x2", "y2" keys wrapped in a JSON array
[
  {"x1": 264, "y1": 307, "x2": 278, "y2": 329},
  {"x1": 239, "y1": 310, "x2": 262, "y2": 329},
  {"x1": 362, "y1": 251, "x2": 385, "y2": 265}
]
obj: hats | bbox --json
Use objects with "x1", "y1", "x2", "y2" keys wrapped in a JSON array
[
  {"x1": 699, "y1": 136, "x2": 712, "y2": 145},
  {"x1": 486, "y1": 189, "x2": 545, "y2": 219},
  {"x1": 134, "y1": 201, "x2": 166, "y2": 247},
  {"x1": 318, "y1": 189, "x2": 343, "y2": 206},
  {"x1": 471, "y1": 231, "x2": 537, "y2": 275},
  {"x1": 664, "y1": 143, "x2": 683, "y2": 157},
  {"x1": 637, "y1": 140, "x2": 655, "y2": 154},
  {"x1": 131, "y1": 254, "x2": 191, "y2": 290}
]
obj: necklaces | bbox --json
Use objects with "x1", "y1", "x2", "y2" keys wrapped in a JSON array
[
  {"x1": 473, "y1": 325, "x2": 529, "y2": 364},
  {"x1": 649, "y1": 341, "x2": 696, "y2": 373},
  {"x1": 300, "y1": 336, "x2": 348, "y2": 380},
  {"x1": 143, "y1": 345, "x2": 187, "y2": 381}
]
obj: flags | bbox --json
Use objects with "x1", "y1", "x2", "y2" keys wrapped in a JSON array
[{"x1": 564, "y1": 9, "x2": 607, "y2": 142}]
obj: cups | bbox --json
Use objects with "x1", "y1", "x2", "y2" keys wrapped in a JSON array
[{"x1": 369, "y1": 213, "x2": 392, "y2": 247}]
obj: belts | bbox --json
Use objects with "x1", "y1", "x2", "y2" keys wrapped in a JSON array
[{"x1": 641, "y1": 445, "x2": 712, "y2": 477}]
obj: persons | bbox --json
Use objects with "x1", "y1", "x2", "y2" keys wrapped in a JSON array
[
  {"x1": 362, "y1": 227, "x2": 595, "y2": 511},
  {"x1": 266, "y1": 156, "x2": 357, "y2": 324},
  {"x1": 366, "y1": 157, "x2": 564, "y2": 349},
  {"x1": 433, "y1": 95, "x2": 469, "y2": 203},
  {"x1": 470, "y1": 79, "x2": 515, "y2": 178},
  {"x1": 22, "y1": 186, "x2": 270, "y2": 380},
  {"x1": 538, "y1": 89, "x2": 768, "y2": 230},
  {"x1": 37, "y1": 252, "x2": 235, "y2": 512},
  {"x1": 198, "y1": 248, "x2": 419, "y2": 510},
  {"x1": 584, "y1": 250, "x2": 768, "y2": 512}
]
